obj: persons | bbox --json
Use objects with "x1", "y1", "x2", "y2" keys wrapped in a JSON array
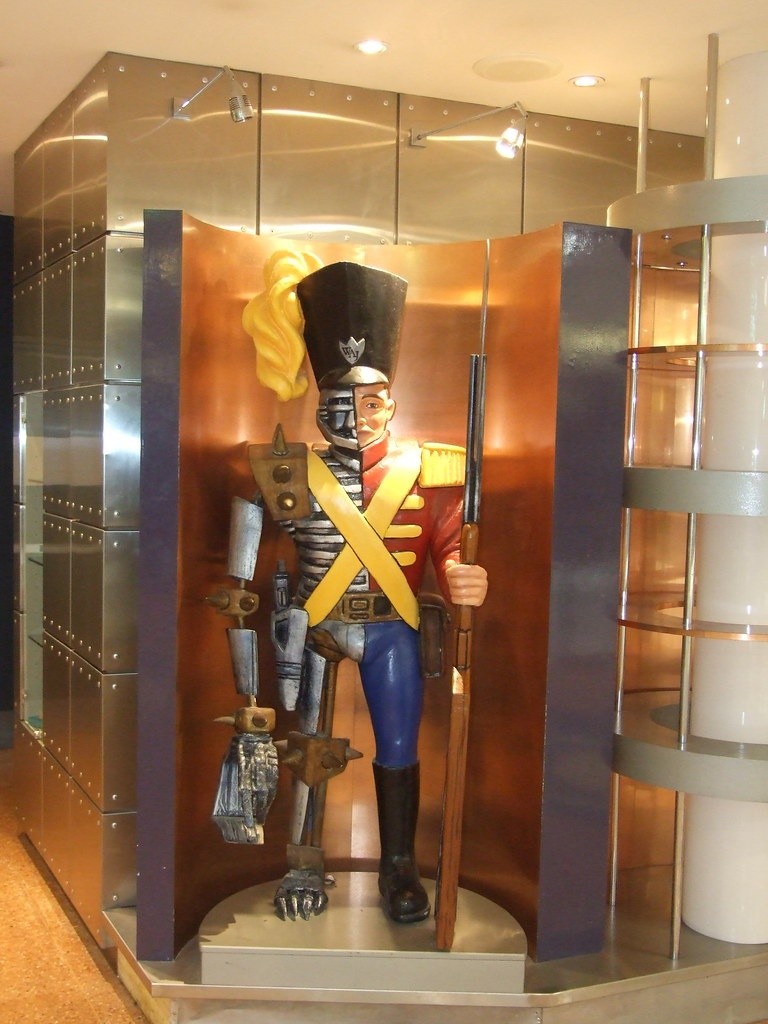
[{"x1": 211, "y1": 262, "x2": 488, "y2": 923}]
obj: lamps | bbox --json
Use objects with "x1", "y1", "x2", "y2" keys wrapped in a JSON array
[
  {"x1": 409, "y1": 100, "x2": 531, "y2": 159},
  {"x1": 171, "y1": 64, "x2": 255, "y2": 124}
]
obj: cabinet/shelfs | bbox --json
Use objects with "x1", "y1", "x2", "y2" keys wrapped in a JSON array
[{"x1": 599, "y1": 31, "x2": 768, "y2": 971}]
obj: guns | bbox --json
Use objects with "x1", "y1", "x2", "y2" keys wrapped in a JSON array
[{"x1": 432, "y1": 351, "x2": 488, "y2": 953}]
